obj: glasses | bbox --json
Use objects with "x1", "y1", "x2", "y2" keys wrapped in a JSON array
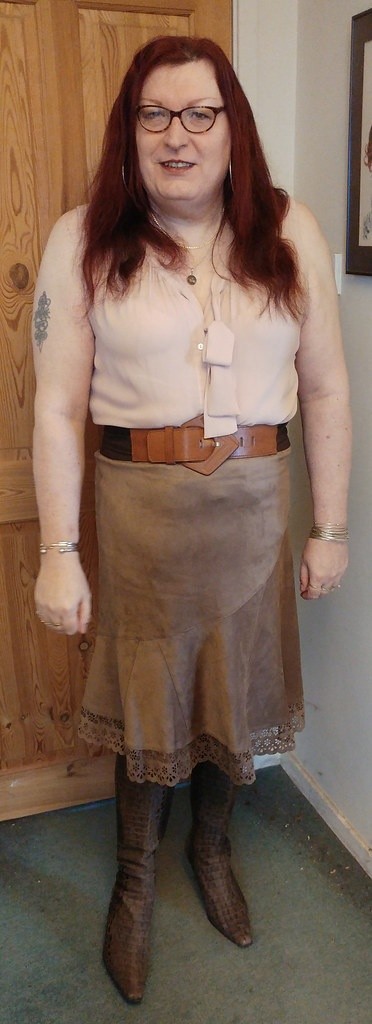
[{"x1": 133, "y1": 103, "x2": 226, "y2": 134}]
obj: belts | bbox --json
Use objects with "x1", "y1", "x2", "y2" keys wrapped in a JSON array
[{"x1": 100, "y1": 414, "x2": 291, "y2": 476}]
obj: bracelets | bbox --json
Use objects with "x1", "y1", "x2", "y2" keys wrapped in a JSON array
[
  {"x1": 37, "y1": 542, "x2": 80, "y2": 552},
  {"x1": 310, "y1": 521, "x2": 349, "y2": 541}
]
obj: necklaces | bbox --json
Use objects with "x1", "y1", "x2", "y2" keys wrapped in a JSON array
[{"x1": 150, "y1": 213, "x2": 218, "y2": 283}]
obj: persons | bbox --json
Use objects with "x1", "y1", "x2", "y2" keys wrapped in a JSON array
[{"x1": 31, "y1": 35, "x2": 352, "y2": 1006}]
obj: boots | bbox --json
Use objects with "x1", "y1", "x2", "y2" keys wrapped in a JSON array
[
  {"x1": 102, "y1": 751, "x2": 174, "y2": 1004},
  {"x1": 185, "y1": 761, "x2": 253, "y2": 949}
]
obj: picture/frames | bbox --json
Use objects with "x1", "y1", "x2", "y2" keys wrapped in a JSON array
[{"x1": 345, "y1": 8, "x2": 372, "y2": 276}]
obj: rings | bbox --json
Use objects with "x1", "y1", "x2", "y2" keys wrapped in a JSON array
[
  {"x1": 309, "y1": 584, "x2": 341, "y2": 591},
  {"x1": 40, "y1": 618, "x2": 62, "y2": 628}
]
